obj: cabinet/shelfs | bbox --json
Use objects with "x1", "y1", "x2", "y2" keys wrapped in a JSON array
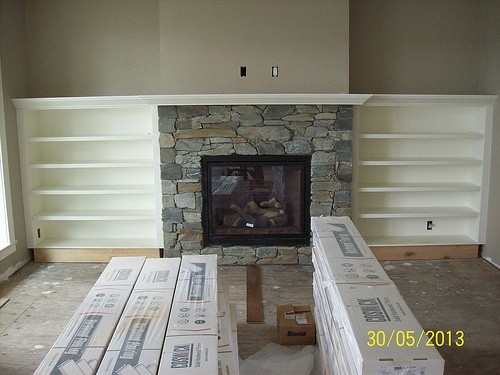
[
  {"x1": 13, "y1": 98, "x2": 165, "y2": 260},
  {"x1": 353, "y1": 94, "x2": 497, "y2": 258}
]
